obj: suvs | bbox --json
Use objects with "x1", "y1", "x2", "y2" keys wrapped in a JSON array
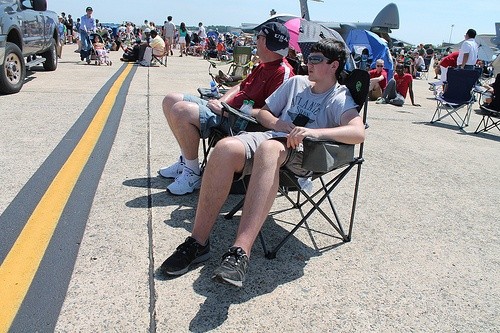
[{"x1": 1, "y1": 0, "x2": 60, "y2": 91}]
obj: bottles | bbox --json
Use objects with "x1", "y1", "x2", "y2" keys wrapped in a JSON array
[
  {"x1": 239, "y1": 100, "x2": 248, "y2": 111},
  {"x1": 232, "y1": 100, "x2": 255, "y2": 133},
  {"x1": 210, "y1": 81, "x2": 219, "y2": 97}
]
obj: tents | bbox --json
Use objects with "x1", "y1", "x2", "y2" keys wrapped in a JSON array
[{"x1": 346, "y1": 28, "x2": 394, "y2": 80}]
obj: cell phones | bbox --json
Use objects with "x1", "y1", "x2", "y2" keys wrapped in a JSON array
[{"x1": 292, "y1": 113, "x2": 310, "y2": 127}]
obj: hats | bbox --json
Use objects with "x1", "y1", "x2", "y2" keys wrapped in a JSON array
[
  {"x1": 260, "y1": 23, "x2": 291, "y2": 57},
  {"x1": 85, "y1": 7, "x2": 93, "y2": 11},
  {"x1": 143, "y1": 28, "x2": 151, "y2": 33}
]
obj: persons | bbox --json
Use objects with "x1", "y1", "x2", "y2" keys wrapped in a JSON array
[
  {"x1": 285, "y1": 45, "x2": 310, "y2": 76},
  {"x1": 439, "y1": 51, "x2": 459, "y2": 93},
  {"x1": 159, "y1": 38, "x2": 366, "y2": 287},
  {"x1": 55, "y1": 6, "x2": 264, "y2": 84},
  {"x1": 456, "y1": 28, "x2": 478, "y2": 69},
  {"x1": 389, "y1": 43, "x2": 494, "y2": 81},
  {"x1": 156, "y1": 22, "x2": 295, "y2": 198},
  {"x1": 354, "y1": 47, "x2": 373, "y2": 73},
  {"x1": 368, "y1": 59, "x2": 388, "y2": 101},
  {"x1": 375, "y1": 63, "x2": 421, "y2": 107}
]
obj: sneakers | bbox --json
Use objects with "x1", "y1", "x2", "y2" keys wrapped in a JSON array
[
  {"x1": 376, "y1": 96, "x2": 387, "y2": 104},
  {"x1": 210, "y1": 247, "x2": 251, "y2": 289},
  {"x1": 159, "y1": 157, "x2": 186, "y2": 177},
  {"x1": 159, "y1": 234, "x2": 210, "y2": 277},
  {"x1": 166, "y1": 168, "x2": 202, "y2": 195}
]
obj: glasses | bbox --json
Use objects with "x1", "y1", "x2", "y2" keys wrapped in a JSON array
[
  {"x1": 307, "y1": 54, "x2": 329, "y2": 64},
  {"x1": 376, "y1": 64, "x2": 384, "y2": 68},
  {"x1": 394, "y1": 69, "x2": 402, "y2": 72}
]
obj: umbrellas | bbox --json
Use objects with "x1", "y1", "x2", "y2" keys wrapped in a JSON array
[
  {"x1": 296, "y1": 17, "x2": 358, "y2": 72},
  {"x1": 475, "y1": 36, "x2": 493, "y2": 61},
  {"x1": 251, "y1": 13, "x2": 306, "y2": 52}
]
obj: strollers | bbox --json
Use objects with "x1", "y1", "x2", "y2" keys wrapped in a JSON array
[{"x1": 85, "y1": 31, "x2": 112, "y2": 67}]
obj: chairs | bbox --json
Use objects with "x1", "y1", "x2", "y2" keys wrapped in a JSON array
[
  {"x1": 224, "y1": 65, "x2": 371, "y2": 260},
  {"x1": 197, "y1": 56, "x2": 301, "y2": 196},
  {"x1": 474, "y1": 73, "x2": 500, "y2": 135},
  {"x1": 430, "y1": 68, "x2": 480, "y2": 130},
  {"x1": 417, "y1": 58, "x2": 432, "y2": 81},
  {"x1": 152, "y1": 41, "x2": 170, "y2": 68}
]
